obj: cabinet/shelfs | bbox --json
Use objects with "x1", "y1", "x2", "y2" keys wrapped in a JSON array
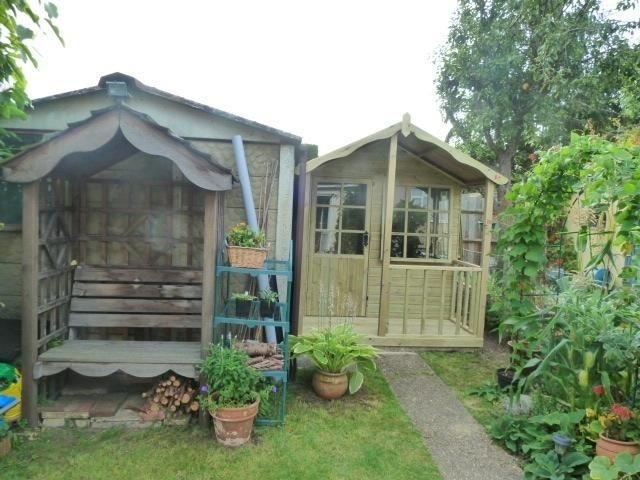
[{"x1": 215, "y1": 261, "x2": 291, "y2": 425}]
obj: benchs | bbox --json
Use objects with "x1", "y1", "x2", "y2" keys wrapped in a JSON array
[{"x1": 34, "y1": 264, "x2": 204, "y2": 398}]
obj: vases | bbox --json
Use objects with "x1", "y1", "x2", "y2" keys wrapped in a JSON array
[
  {"x1": 595, "y1": 431, "x2": 639, "y2": 464},
  {"x1": 496, "y1": 341, "x2": 541, "y2": 390}
]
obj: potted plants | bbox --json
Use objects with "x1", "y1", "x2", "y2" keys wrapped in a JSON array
[{"x1": 196, "y1": 323, "x2": 383, "y2": 448}]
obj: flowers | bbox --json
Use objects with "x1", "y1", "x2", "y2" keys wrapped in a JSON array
[
  {"x1": 223, "y1": 222, "x2": 271, "y2": 249},
  {"x1": 587, "y1": 382, "x2": 639, "y2": 442}
]
